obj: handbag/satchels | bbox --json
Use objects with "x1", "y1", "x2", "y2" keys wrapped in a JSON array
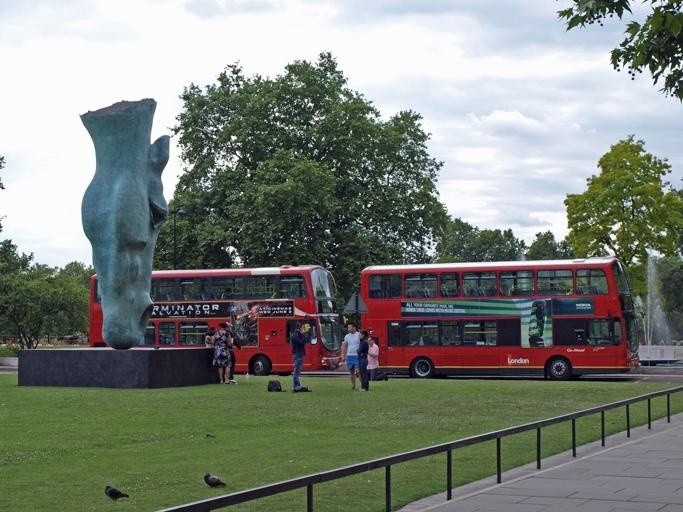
[{"x1": 267, "y1": 381, "x2": 282, "y2": 391}]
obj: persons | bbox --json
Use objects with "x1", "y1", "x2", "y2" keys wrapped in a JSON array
[
  {"x1": 355, "y1": 330, "x2": 370, "y2": 392},
  {"x1": 146, "y1": 332, "x2": 194, "y2": 345},
  {"x1": 289, "y1": 320, "x2": 309, "y2": 392},
  {"x1": 211, "y1": 323, "x2": 233, "y2": 386},
  {"x1": 339, "y1": 323, "x2": 361, "y2": 391},
  {"x1": 225, "y1": 321, "x2": 238, "y2": 384},
  {"x1": 204, "y1": 327, "x2": 218, "y2": 349},
  {"x1": 368, "y1": 337, "x2": 388, "y2": 382}
]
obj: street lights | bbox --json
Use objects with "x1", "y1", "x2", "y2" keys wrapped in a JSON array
[{"x1": 168, "y1": 209, "x2": 185, "y2": 270}]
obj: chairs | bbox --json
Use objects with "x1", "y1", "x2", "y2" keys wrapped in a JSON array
[
  {"x1": 154, "y1": 332, "x2": 196, "y2": 344},
  {"x1": 404, "y1": 333, "x2": 478, "y2": 345},
  {"x1": 370, "y1": 282, "x2": 604, "y2": 297},
  {"x1": 149, "y1": 289, "x2": 227, "y2": 300}
]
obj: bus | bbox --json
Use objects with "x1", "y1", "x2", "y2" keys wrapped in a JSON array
[
  {"x1": 87, "y1": 264, "x2": 344, "y2": 376},
  {"x1": 359, "y1": 257, "x2": 639, "y2": 381}
]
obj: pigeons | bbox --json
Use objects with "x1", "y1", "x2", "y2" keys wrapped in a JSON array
[
  {"x1": 204, "y1": 472, "x2": 228, "y2": 489},
  {"x1": 103, "y1": 484, "x2": 131, "y2": 502}
]
